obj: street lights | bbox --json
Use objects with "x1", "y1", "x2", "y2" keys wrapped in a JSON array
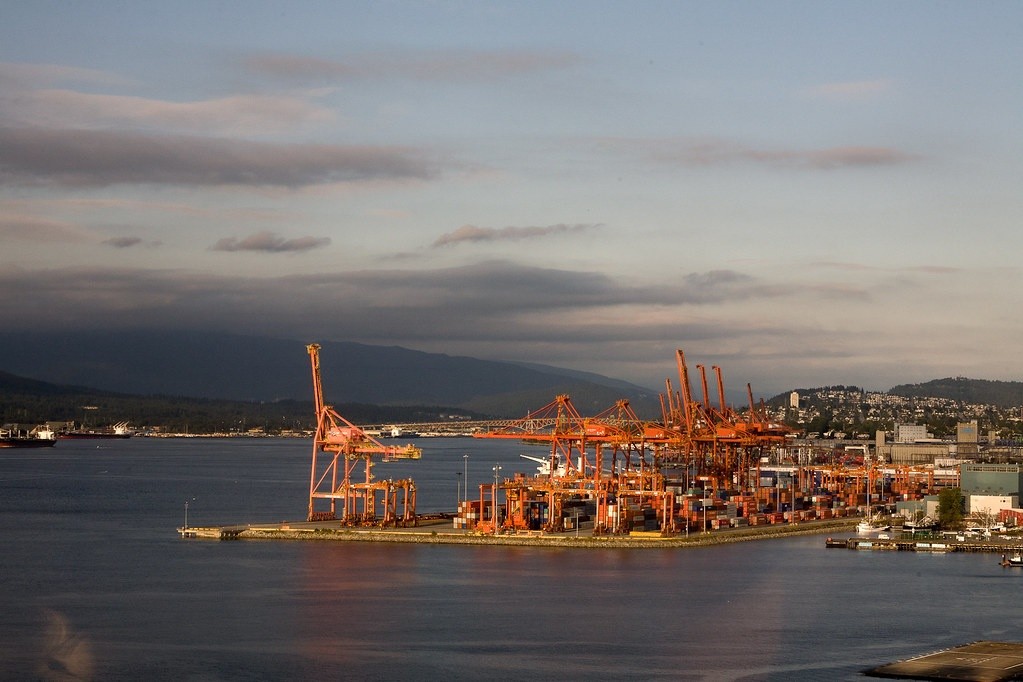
[
  {"x1": 456, "y1": 472, "x2": 462, "y2": 501},
  {"x1": 492, "y1": 464, "x2": 502, "y2": 534},
  {"x1": 463, "y1": 455, "x2": 468, "y2": 501}
]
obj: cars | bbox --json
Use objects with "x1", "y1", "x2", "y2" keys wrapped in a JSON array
[{"x1": 879, "y1": 534, "x2": 889, "y2": 540}]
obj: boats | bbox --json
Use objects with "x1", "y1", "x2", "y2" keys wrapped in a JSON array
[
  {"x1": 0, "y1": 425, "x2": 57, "y2": 447},
  {"x1": 856, "y1": 521, "x2": 890, "y2": 532},
  {"x1": 394, "y1": 430, "x2": 420, "y2": 438},
  {"x1": 902, "y1": 524, "x2": 933, "y2": 533}
]
